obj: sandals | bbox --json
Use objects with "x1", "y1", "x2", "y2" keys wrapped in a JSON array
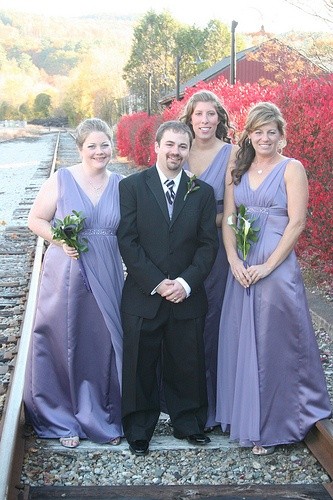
[
  {"x1": 252, "y1": 444, "x2": 276, "y2": 455},
  {"x1": 107, "y1": 437, "x2": 121, "y2": 446},
  {"x1": 59, "y1": 435, "x2": 79, "y2": 448}
]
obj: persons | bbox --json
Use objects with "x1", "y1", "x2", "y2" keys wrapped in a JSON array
[
  {"x1": 22, "y1": 118, "x2": 127, "y2": 447},
  {"x1": 214, "y1": 100, "x2": 332, "y2": 454},
  {"x1": 179, "y1": 90, "x2": 237, "y2": 431},
  {"x1": 117, "y1": 121, "x2": 219, "y2": 455}
]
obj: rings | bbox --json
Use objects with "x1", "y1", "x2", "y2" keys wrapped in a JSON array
[
  {"x1": 174, "y1": 298, "x2": 176, "y2": 301},
  {"x1": 236, "y1": 276, "x2": 239, "y2": 278}
]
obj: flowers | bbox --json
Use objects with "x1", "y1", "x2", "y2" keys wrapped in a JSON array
[
  {"x1": 50, "y1": 210, "x2": 88, "y2": 294},
  {"x1": 184, "y1": 173, "x2": 200, "y2": 202},
  {"x1": 227, "y1": 203, "x2": 261, "y2": 297}
]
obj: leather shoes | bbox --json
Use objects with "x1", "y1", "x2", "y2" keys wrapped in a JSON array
[
  {"x1": 128, "y1": 439, "x2": 149, "y2": 455},
  {"x1": 174, "y1": 432, "x2": 211, "y2": 445}
]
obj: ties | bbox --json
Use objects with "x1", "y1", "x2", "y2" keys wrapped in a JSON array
[{"x1": 165, "y1": 179, "x2": 175, "y2": 205}]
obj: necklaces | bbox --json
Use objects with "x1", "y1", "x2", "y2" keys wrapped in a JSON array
[
  {"x1": 257, "y1": 157, "x2": 276, "y2": 174},
  {"x1": 92, "y1": 184, "x2": 102, "y2": 197}
]
